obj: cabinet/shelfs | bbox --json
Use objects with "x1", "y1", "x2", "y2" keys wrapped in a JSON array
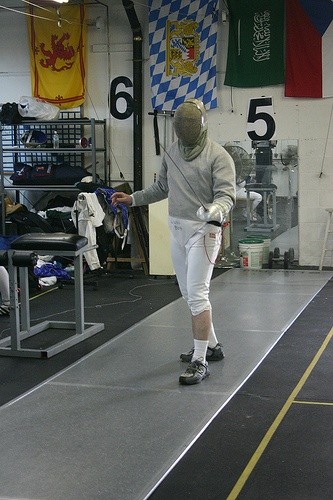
[{"x1": 0, "y1": 117, "x2": 107, "y2": 237}]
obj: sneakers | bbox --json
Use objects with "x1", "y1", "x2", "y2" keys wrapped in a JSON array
[
  {"x1": 179, "y1": 356, "x2": 210, "y2": 384},
  {"x1": 180, "y1": 342, "x2": 224, "y2": 362}
]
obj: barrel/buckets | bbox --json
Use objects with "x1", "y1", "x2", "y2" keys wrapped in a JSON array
[
  {"x1": 238, "y1": 239, "x2": 264, "y2": 270},
  {"x1": 247, "y1": 235, "x2": 271, "y2": 264}
]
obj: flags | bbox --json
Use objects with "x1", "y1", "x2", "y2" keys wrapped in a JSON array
[
  {"x1": 25, "y1": 0, "x2": 87, "y2": 109},
  {"x1": 223, "y1": 0, "x2": 285, "y2": 88},
  {"x1": 147, "y1": 0, "x2": 219, "y2": 112},
  {"x1": 287, "y1": 0, "x2": 333, "y2": 98}
]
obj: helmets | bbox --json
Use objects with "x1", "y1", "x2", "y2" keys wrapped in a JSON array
[{"x1": 173, "y1": 99, "x2": 208, "y2": 162}]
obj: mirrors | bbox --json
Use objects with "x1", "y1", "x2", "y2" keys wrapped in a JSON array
[{"x1": 217, "y1": 139, "x2": 299, "y2": 270}]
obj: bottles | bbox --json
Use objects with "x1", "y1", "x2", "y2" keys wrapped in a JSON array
[{"x1": 52, "y1": 131, "x2": 59, "y2": 148}]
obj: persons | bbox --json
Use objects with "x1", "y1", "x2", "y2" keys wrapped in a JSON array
[
  {"x1": 236, "y1": 175, "x2": 262, "y2": 221},
  {"x1": 111, "y1": 99, "x2": 235, "y2": 384}
]
